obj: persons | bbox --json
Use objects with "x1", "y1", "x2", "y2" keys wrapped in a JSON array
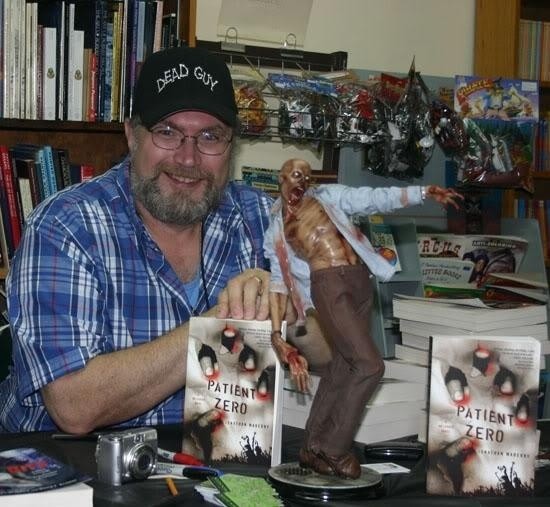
[
  {"x1": 0, "y1": 46, "x2": 333, "y2": 435},
  {"x1": 261, "y1": 158, "x2": 467, "y2": 480}
]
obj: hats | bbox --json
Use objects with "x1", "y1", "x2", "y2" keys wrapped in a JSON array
[{"x1": 131, "y1": 47, "x2": 235, "y2": 129}]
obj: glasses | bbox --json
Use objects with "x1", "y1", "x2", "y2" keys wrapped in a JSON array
[{"x1": 134, "y1": 124, "x2": 234, "y2": 155}]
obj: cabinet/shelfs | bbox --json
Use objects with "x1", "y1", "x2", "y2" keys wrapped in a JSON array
[
  {"x1": 473, "y1": 0, "x2": 549, "y2": 267},
  {"x1": 2, "y1": 0, "x2": 196, "y2": 280}
]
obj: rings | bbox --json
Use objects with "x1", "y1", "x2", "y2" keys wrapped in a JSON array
[{"x1": 251, "y1": 276, "x2": 262, "y2": 287}]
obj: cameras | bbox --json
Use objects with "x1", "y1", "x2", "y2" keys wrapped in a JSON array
[{"x1": 95, "y1": 428, "x2": 158, "y2": 487}]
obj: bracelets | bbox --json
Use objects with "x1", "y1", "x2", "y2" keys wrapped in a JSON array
[
  {"x1": 420, "y1": 185, "x2": 427, "y2": 201},
  {"x1": 271, "y1": 330, "x2": 283, "y2": 336}
]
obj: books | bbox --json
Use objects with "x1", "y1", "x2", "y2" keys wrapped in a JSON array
[
  {"x1": 0, "y1": 142, "x2": 95, "y2": 268},
  {"x1": 284, "y1": 232, "x2": 549, "y2": 496},
  {"x1": 180, "y1": 317, "x2": 288, "y2": 468},
  {"x1": 0, "y1": 0, "x2": 188, "y2": 123},
  {"x1": 0, "y1": 449, "x2": 94, "y2": 506}
]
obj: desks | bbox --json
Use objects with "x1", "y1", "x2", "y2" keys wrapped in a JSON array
[{"x1": 2, "y1": 419, "x2": 548, "y2": 505}]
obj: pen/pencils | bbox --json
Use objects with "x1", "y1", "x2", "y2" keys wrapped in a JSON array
[
  {"x1": 155, "y1": 462, "x2": 224, "y2": 478},
  {"x1": 166, "y1": 478, "x2": 179, "y2": 497},
  {"x1": 158, "y1": 447, "x2": 211, "y2": 466}
]
